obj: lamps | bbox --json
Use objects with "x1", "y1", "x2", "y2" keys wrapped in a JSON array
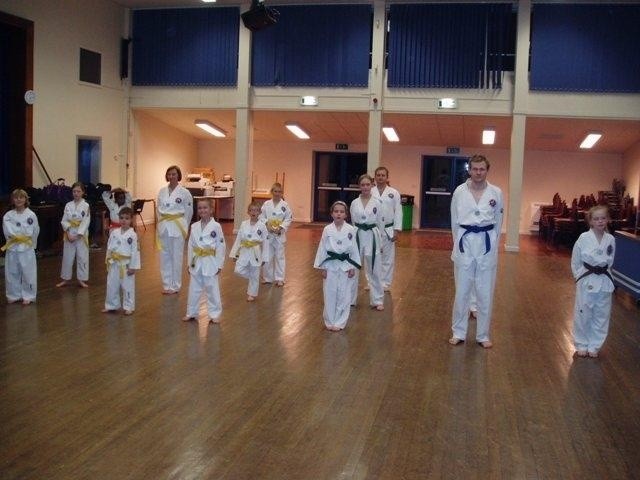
[
  {"x1": 382, "y1": 123, "x2": 402, "y2": 143},
  {"x1": 283, "y1": 120, "x2": 312, "y2": 140},
  {"x1": 482, "y1": 126, "x2": 496, "y2": 146},
  {"x1": 193, "y1": 118, "x2": 227, "y2": 138},
  {"x1": 576, "y1": 130, "x2": 602, "y2": 151}
]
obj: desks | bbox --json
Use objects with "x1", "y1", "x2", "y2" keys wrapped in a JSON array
[{"x1": 193, "y1": 195, "x2": 234, "y2": 222}]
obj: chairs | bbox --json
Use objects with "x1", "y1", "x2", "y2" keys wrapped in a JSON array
[
  {"x1": 0, "y1": 197, "x2": 109, "y2": 250},
  {"x1": 537, "y1": 176, "x2": 640, "y2": 252}
]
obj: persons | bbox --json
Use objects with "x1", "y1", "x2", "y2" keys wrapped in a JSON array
[
  {"x1": 468, "y1": 284, "x2": 476, "y2": 319},
  {"x1": 182, "y1": 198, "x2": 226, "y2": 323},
  {"x1": 364, "y1": 167, "x2": 402, "y2": 292},
  {"x1": 350, "y1": 174, "x2": 385, "y2": 311},
  {"x1": 102, "y1": 208, "x2": 141, "y2": 315},
  {"x1": 258, "y1": 183, "x2": 293, "y2": 286},
  {"x1": 102, "y1": 188, "x2": 131, "y2": 237},
  {"x1": 228, "y1": 202, "x2": 269, "y2": 301},
  {"x1": 571, "y1": 206, "x2": 616, "y2": 357},
  {"x1": 1, "y1": 189, "x2": 40, "y2": 304},
  {"x1": 156, "y1": 165, "x2": 194, "y2": 295},
  {"x1": 313, "y1": 201, "x2": 361, "y2": 331},
  {"x1": 55, "y1": 182, "x2": 90, "y2": 288},
  {"x1": 449, "y1": 156, "x2": 504, "y2": 348}
]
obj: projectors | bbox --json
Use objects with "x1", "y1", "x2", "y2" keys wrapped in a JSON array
[{"x1": 241, "y1": 6, "x2": 280, "y2": 31}]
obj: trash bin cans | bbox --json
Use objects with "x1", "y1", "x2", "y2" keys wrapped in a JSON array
[{"x1": 400, "y1": 194, "x2": 414, "y2": 231}]
